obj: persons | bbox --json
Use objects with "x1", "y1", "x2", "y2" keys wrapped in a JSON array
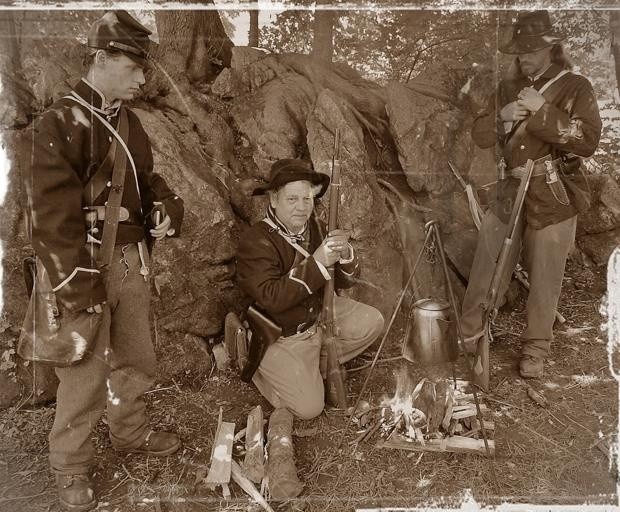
[
  {"x1": 16, "y1": 11, "x2": 185, "y2": 511},
  {"x1": 453, "y1": 9, "x2": 602, "y2": 380},
  {"x1": 221, "y1": 158, "x2": 386, "y2": 420}
]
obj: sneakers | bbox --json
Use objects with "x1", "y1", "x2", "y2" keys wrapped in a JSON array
[
  {"x1": 55, "y1": 472, "x2": 99, "y2": 512},
  {"x1": 519, "y1": 354, "x2": 545, "y2": 380},
  {"x1": 132, "y1": 429, "x2": 182, "y2": 459}
]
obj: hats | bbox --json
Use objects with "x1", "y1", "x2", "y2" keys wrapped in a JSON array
[
  {"x1": 497, "y1": 9, "x2": 569, "y2": 56},
  {"x1": 87, "y1": 9, "x2": 157, "y2": 71},
  {"x1": 250, "y1": 158, "x2": 331, "y2": 200}
]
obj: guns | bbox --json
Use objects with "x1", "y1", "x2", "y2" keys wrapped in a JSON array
[
  {"x1": 241, "y1": 306, "x2": 282, "y2": 383},
  {"x1": 468, "y1": 158, "x2": 534, "y2": 394},
  {"x1": 318, "y1": 129, "x2": 347, "y2": 409},
  {"x1": 448, "y1": 160, "x2": 566, "y2": 327}
]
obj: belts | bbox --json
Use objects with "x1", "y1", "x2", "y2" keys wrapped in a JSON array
[
  {"x1": 509, "y1": 153, "x2": 571, "y2": 180},
  {"x1": 281, "y1": 319, "x2": 317, "y2": 337},
  {"x1": 83, "y1": 206, "x2": 142, "y2": 223}
]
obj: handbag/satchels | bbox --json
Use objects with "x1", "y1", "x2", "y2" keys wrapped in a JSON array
[
  {"x1": 16, "y1": 263, "x2": 108, "y2": 367},
  {"x1": 223, "y1": 311, "x2": 251, "y2": 372},
  {"x1": 558, "y1": 155, "x2": 593, "y2": 214}
]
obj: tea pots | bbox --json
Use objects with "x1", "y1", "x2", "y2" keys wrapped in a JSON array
[{"x1": 400, "y1": 279, "x2": 460, "y2": 367}]
